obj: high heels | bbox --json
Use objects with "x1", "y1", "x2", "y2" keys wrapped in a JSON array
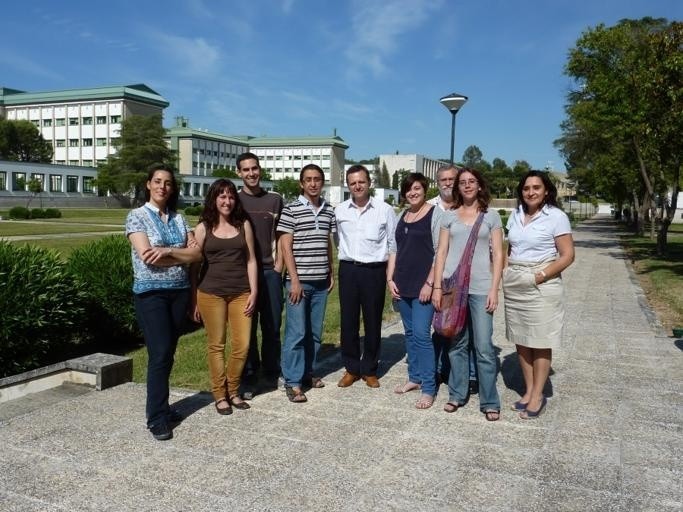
[{"x1": 511, "y1": 396, "x2": 547, "y2": 419}]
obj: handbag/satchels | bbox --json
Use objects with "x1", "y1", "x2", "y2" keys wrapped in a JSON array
[{"x1": 433, "y1": 263, "x2": 471, "y2": 337}]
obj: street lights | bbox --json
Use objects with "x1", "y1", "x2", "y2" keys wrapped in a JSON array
[
  {"x1": 437, "y1": 91, "x2": 469, "y2": 169},
  {"x1": 561, "y1": 181, "x2": 598, "y2": 221}
]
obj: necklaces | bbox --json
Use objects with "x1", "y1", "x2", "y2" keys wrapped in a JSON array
[{"x1": 403, "y1": 202, "x2": 425, "y2": 235}]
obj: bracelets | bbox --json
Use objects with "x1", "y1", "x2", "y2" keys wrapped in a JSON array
[
  {"x1": 427, "y1": 279, "x2": 433, "y2": 287},
  {"x1": 387, "y1": 278, "x2": 392, "y2": 284},
  {"x1": 434, "y1": 287, "x2": 442, "y2": 291},
  {"x1": 168, "y1": 247, "x2": 173, "y2": 257},
  {"x1": 541, "y1": 270, "x2": 550, "y2": 283}
]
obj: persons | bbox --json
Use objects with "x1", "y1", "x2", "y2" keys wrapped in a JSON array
[
  {"x1": 276, "y1": 164, "x2": 337, "y2": 403},
  {"x1": 436, "y1": 166, "x2": 506, "y2": 422},
  {"x1": 236, "y1": 151, "x2": 287, "y2": 400},
  {"x1": 122, "y1": 164, "x2": 203, "y2": 442},
  {"x1": 190, "y1": 178, "x2": 258, "y2": 417},
  {"x1": 502, "y1": 171, "x2": 577, "y2": 421},
  {"x1": 333, "y1": 163, "x2": 395, "y2": 388},
  {"x1": 385, "y1": 172, "x2": 447, "y2": 411},
  {"x1": 425, "y1": 164, "x2": 480, "y2": 393}
]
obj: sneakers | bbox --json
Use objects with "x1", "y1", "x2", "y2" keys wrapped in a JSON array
[
  {"x1": 150, "y1": 423, "x2": 171, "y2": 440},
  {"x1": 163, "y1": 406, "x2": 184, "y2": 421},
  {"x1": 244, "y1": 361, "x2": 324, "y2": 402}
]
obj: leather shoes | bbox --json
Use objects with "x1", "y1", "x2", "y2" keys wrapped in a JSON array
[
  {"x1": 467, "y1": 380, "x2": 478, "y2": 395},
  {"x1": 360, "y1": 371, "x2": 380, "y2": 388},
  {"x1": 215, "y1": 398, "x2": 233, "y2": 414},
  {"x1": 338, "y1": 372, "x2": 359, "y2": 388},
  {"x1": 229, "y1": 394, "x2": 250, "y2": 409}
]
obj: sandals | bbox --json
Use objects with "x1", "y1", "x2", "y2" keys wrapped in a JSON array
[
  {"x1": 445, "y1": 402, "x2": 462, "y2": 413},
  {"x1": 485, "y1": 409, "x2": 499, "y2": 420},
  {"x1": 394, "y1": 382, "x2": 422, "y2": 394},
  {"x1": 414, "y1": 394, "x2": 434, "y2": 408}
]
